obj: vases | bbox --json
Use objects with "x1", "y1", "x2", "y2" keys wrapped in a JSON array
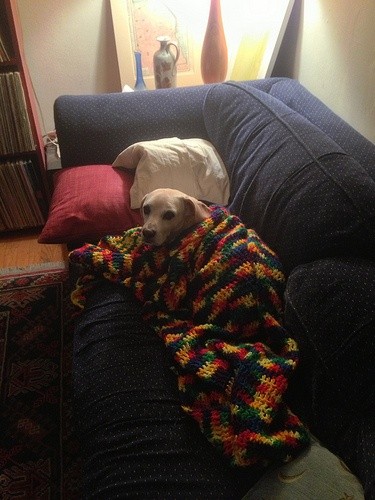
[
  {"x1": 201, "y1": 1, "x2": 227, "y2": 84},
  {"x1": 153, "y1": 36, "x2": 180, "y2": 89},
  {"x1": 132, "y1": 52, "x2": 147, "y2": 92}
]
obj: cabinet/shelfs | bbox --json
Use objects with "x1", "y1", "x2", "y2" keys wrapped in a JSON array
[{"x1": 0, "y1": 1, "x2": 51, "y2": 239}]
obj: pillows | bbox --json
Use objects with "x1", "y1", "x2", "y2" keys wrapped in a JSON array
[
  {"x1": 37, "y1": 166, "x2": 143, "y2": 245},
  {"x1": 113, "y1": 138, "x2": 232, "y2": 207}
]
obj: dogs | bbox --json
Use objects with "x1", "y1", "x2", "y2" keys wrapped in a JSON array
[{"x1": 136, "y1": 186, "x2": 216, "y2": 250}]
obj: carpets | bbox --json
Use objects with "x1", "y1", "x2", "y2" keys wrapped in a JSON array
[{"x1": 0, "y1": 260, "x2": 79, "y2": 500}]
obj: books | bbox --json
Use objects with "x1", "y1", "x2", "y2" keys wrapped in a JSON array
[
  {"x1": 1, "y1": 25, "x2": 14, "y2": 64},
  {"x1": 0, "y1": 158, "x2": 46, "y2": 233},
  {"x1": 0, "y1": 70, "x2": 36, "y2": 157}
]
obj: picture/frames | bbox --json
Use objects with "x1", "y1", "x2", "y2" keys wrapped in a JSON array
[{"x1": 109, "y1": 0, "x2": 201, "y2": 91}]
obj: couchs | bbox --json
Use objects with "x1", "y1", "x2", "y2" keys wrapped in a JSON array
[{"x1": 53, "y1": 76, "x2": 375, "y2": 500}]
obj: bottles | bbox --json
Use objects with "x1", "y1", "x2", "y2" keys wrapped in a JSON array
[
  {"x1": 201, "y1": 0, "x2": 228, "y2": 84},
  {"x1": 134, "y1": 51, "x2": 148, "y2": 90},
  {"x1": 153, "y1": 36, "x2": 180, "y2": 89}
]
